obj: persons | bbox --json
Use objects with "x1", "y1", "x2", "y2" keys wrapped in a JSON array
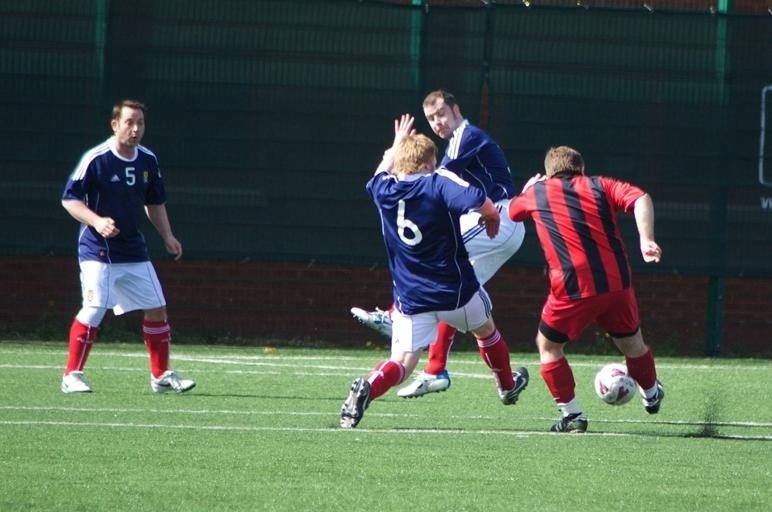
[
  {"x1": 339, "y1": 113, "x2": 529, "y2": 432},
  {"x1": 509, "y1": 144, "x2": 665, "y2": 435},
  {"x1": 58, "y1": 98, "x2": 198, "y2": 395},
  {"x1": 350, "y1": 89, "x2": 525, "y2": 402}
]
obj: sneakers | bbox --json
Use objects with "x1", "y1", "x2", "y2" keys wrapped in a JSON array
[
  {"x1": 61, "y1": 369, "x2": 92, "y2": 394},
  {"x1": 349, "y1": 305, "x2": 394, "y2": 339},
  {"x1": 498, "y1": 366, "x2": 530, "y2": 406},
  {"x1": 151, "y1": 368, "x2": 197, "y2": 395},
  {"x1": 641, "y1": 379, "x2": 665, "y2": 415},
  {"x1": 338, "y1": 377, "x2": 371, "y2": 430},
  {"x1": 550, "y1": 413, "x2": 588, "y2": 434},
  {"x1": 397, "y1": 370, "x2": 451, "y2": 397}
]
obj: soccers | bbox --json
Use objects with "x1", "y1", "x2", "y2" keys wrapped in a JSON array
[{"x1": 595, "y1": 363, "x2": 637, "y2": 406}]
